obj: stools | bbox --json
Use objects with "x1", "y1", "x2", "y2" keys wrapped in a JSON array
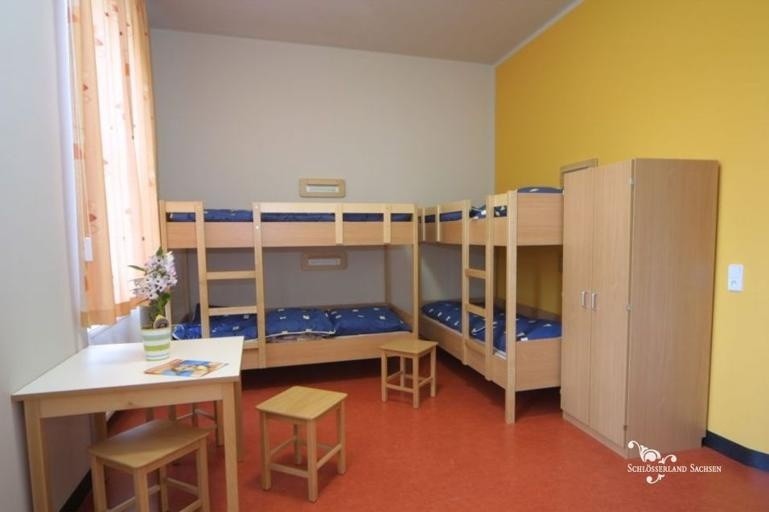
[{"x1": 89, "y1": 382, "x2": 350, "y2": 510}]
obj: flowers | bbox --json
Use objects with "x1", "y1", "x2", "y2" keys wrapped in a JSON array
[{"x1": 130, "y1": 247, "x2": 179, "y2": 325}]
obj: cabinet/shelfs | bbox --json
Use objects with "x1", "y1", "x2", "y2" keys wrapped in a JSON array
[{"x1": 560, "y1": 158, "x2": 718, "y2": 460}]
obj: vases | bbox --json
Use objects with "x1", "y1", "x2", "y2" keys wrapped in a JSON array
[{"x1": 142, "y1": 324, "x2": 172, "y2": 358}]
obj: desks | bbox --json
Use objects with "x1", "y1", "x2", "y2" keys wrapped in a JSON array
[{"x1": 11, "y1": 335, "x2": 244, "y2": 511}]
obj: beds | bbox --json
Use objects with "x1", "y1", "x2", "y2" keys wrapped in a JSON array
[{"x1": 159, "y1": 190, "x2": 562, "y2": 426}]
